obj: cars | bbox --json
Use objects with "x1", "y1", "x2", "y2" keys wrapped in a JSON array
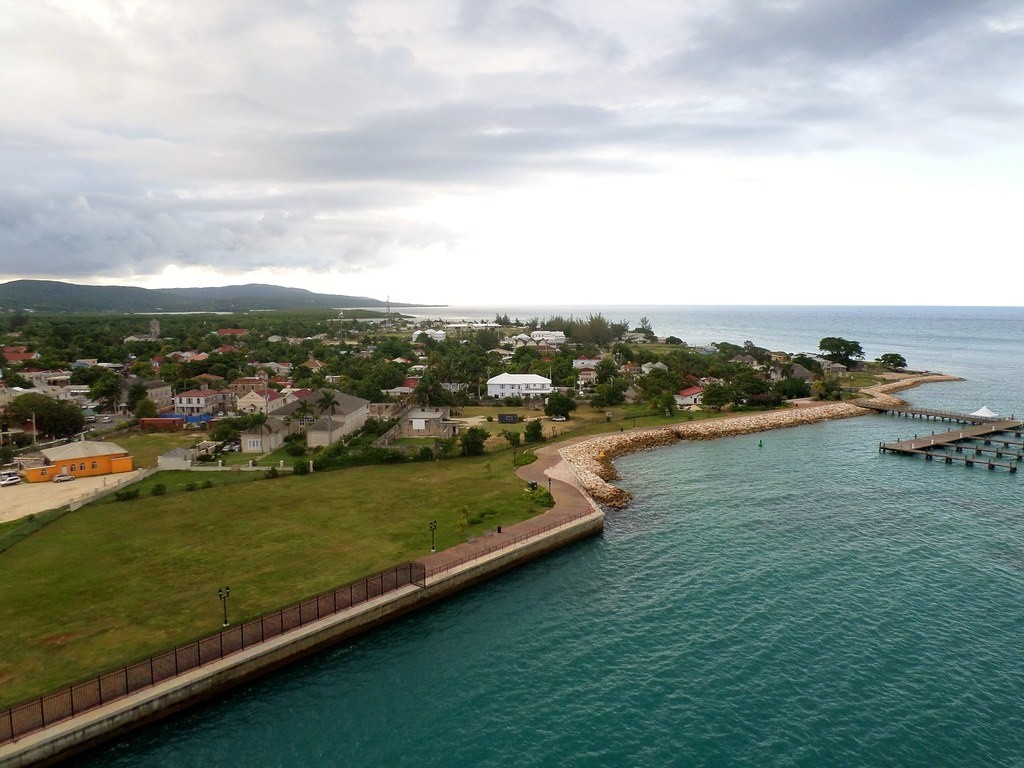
[
  {"x1": 102, "y1": 417, "x2": 112, "y2": 423},
  {"x1": 85, "y1": 416, "x2": 95, "y2": 423},
  {"x1": 52, "y1": 473, "x2": 76, "y2": 483},
  {"x1": 0, "y1": 475, "x2": 22, "y2": 487},
  {"x1": 552, "y1": 416, "x2": 566, "y2": 422}
]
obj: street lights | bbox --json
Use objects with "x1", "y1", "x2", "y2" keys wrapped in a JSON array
[
  {"x1": 512, "y1": 448, "x2": 516, "y2": 468},
  {"x1": 632, "y1": 414, "x2": 637, "y2": 428},
  {"x1": 429, "y1": 520, "x2": 437, "y2": 552},
  {"x1": 27, "y1": 412, "x2": 36, "y2": 445},
  {"x1": 218, "y1": 586, "x2": 231, "y2": 625},
  {"x1": 552, "y1": 426, "x2": 556, "y2": 442}
]
